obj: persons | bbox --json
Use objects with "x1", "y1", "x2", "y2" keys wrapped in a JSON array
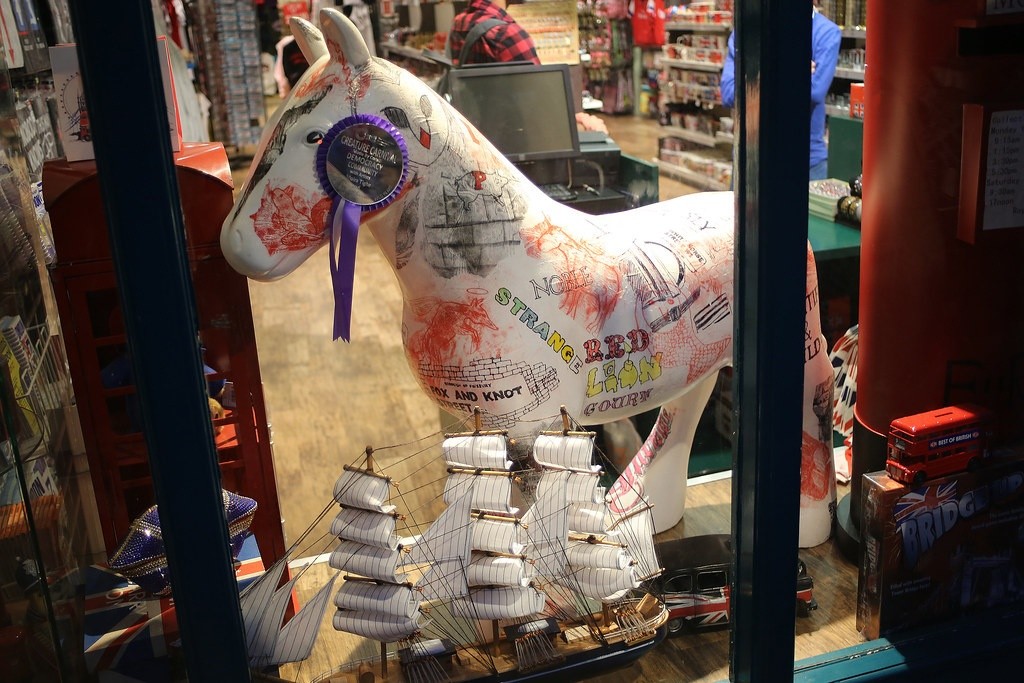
[
  {"x1": 449, "y1": 1, "x2": 611, "y2": 140},
  {"x1": 719, "y1": 3, "x2": 842, "y2": 181}
]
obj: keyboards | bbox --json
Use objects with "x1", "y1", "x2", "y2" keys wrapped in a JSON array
[{"x1": 536, "y1": 183, "x2": 576, "y2": 199}]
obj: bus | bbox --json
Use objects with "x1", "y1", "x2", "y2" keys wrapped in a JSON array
[{"x1": 884, "y1": 397, "x2": 997, "y2": 489}]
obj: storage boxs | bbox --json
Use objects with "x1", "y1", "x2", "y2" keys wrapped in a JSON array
[
  {"x1": 856, "y1": 445, "x2": 1024, "y2": 641},
  {"x1": 84, "y1": 531, "x2": 280, "y2": 683}
]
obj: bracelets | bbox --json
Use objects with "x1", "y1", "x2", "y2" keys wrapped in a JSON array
[{"x1": 578, "y1": 119, "x2": 588, "y2": 131}]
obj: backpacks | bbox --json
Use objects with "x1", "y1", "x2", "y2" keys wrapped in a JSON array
[{"x1": 435, "y1": 19, "x2": 508, "y2": 104}]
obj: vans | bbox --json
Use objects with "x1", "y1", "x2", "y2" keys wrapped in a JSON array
[{"x1": 659, "y1": 553, "x2": 821, "y2": 634}]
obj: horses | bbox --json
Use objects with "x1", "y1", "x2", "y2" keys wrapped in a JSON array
[{"x1": 217, "y1": 7, "x2": 835, "y2": 550}]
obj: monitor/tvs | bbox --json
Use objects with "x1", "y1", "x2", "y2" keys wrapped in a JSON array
[{"x1": 451, "y1": 64, "x2": 580, "y2": 160}]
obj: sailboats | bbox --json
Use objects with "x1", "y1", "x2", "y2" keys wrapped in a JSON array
[{"x1": 239, "y1": 407, "x2": 671, "y2": 683}]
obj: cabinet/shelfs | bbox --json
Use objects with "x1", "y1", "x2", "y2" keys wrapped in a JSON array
[{"x1": 650, "y1": 0, "x2": 866, "y2": 190}]
